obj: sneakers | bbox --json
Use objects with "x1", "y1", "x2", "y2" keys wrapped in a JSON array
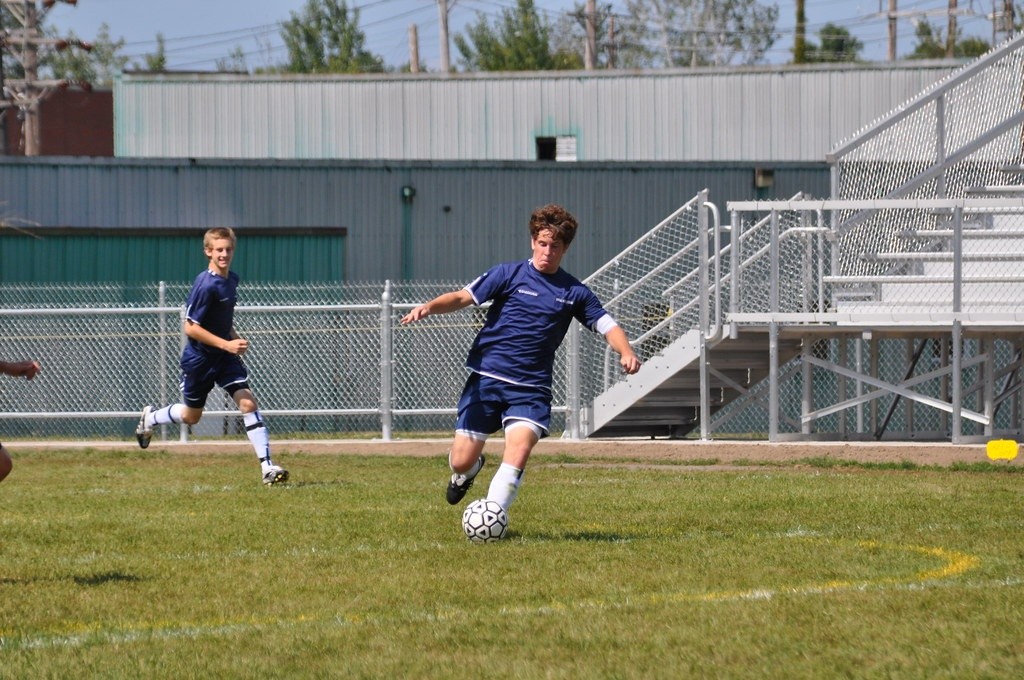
[
  {"x1": 262, "y1": 465, "x2": 289, "y2": 486},
  {"x1": 136, "y1": 405, "x2": 160, "y2": 449},
  {"x1": 446, "y1": 455, "x2": 485, "y2": 505}
]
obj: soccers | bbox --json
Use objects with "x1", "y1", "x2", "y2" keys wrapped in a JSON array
[{"x1": 461, "y1": 499, "x2": 508, "y2": 544}]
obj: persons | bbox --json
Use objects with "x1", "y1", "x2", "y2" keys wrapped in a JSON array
[
  {"x1": 0, "y1": 361, "x2": 41, "y2": 481},
  {"x1": 401, "y1": 203, "x2": 640, "y2": 512},
  {"x1": 135, "y1": 227, "x2": 290, "y2": 487}
]
